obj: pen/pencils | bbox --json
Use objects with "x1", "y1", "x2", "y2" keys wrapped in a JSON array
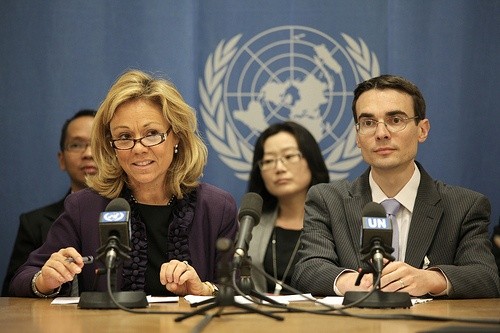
[{"x1": 64, "y1": 254, "x2": 94, "y2": 265}]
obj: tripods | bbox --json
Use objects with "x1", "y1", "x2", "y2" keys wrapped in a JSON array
[{"x1": 176, "y1": 256, "x2": 286, "y2": 333}]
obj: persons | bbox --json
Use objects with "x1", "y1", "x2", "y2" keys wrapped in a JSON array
[
  {"x1": 9, "y1": 69, "x2": 239, "y2": 299},
  {"x1": 291, "y1": 74, "x2": 500, "y2": 298},
  {"x1": 1, "y1": 109, "x2": 99, "y2": 297},
  {"x1": 248, "y1": 121, "x2": 330, "y2": 295}
]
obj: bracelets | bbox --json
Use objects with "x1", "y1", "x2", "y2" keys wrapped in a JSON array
[{"x1": 32, "y1": 271, "x2": 61, "y2": 297}]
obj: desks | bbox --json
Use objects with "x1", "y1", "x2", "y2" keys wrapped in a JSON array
[{"x1": 0, "y1": 297, "x2": 500, "y2": 333}]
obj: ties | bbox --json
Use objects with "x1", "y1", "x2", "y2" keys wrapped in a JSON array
[{"x1": 381, "y1": 198, "x2": 401, "y2": 261}]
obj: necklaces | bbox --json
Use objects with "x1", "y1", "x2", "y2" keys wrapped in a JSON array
[{"x1": 272, "y1": 231, "x2": 302, "y2": 294}]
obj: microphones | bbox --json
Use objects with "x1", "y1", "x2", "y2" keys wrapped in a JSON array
[
  {"x1": 358, "y1": 201, "x2": 393, "y2": 271},
  {"x1": 97, "y1": 198, "x2": 132, "y2": 268},
  {"x1": 232, "y1": 192, "x2": 265, "y2": 269}
]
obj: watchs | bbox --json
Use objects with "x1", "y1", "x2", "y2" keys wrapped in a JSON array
[{"x1": 203, "y1": 281, "x2": 219, "y2": 296}]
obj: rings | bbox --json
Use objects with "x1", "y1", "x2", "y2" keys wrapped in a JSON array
[
  {"x1": 183, "y1": 261, "x2": 188, "y2": 270},
  {"x1": 398, "y1": 280, "x2": 404, "y2": 288}
]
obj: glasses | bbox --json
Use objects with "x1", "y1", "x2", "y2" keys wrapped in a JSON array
[
  {"x1": 257, "y1": 152, "x2": 303, "y2": 170},
  {"x1": 64, "y1": 141, "x2": 91, "y2": 153},
  {"x1": 355, "y1": 114, "x2": 418, "y2": 135},
  {"x1": 109, "y1": 125, "x2": 172, "y2": 150}
]
